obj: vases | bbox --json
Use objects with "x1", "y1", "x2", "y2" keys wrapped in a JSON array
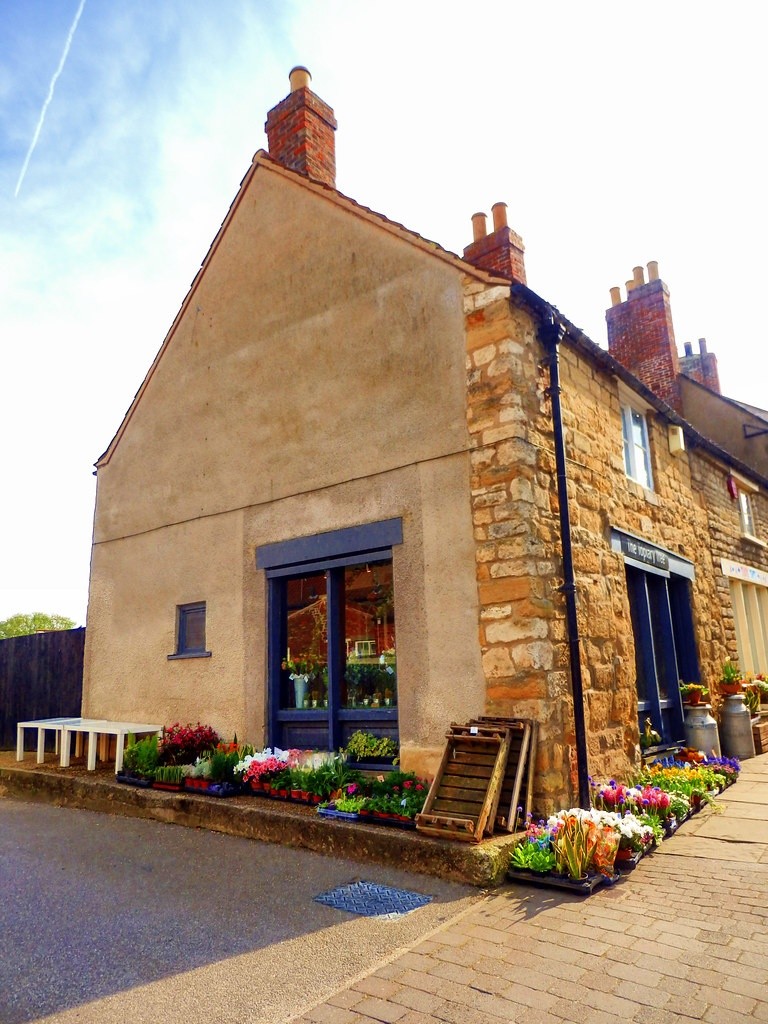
[
  {"x1": 294, "y1": 678, "x2": 309, "y2": 707},
  {"x1": 551, "y1": 785, "x2": 710, "y2": 900}
]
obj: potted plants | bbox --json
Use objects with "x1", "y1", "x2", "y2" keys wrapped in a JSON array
[
  {"x1": 509, "y1": 842, "x2": 557, "y2": 877},
  {"x1": 679, "y1": 680, "x2": 709, "y2": 704},
  {"x1": 115, "y1": 732, "x2": 160, "y2": 787},
  {"x1": 740, "y1": 689, "x2": 761, "y2": 720},
  {"x1": 719, "y1": 665, "x2": 742, "y2": 694},
  {"x1": 337, "y1": 730, "x2": 399, "y2": 765},
  {"x1": 154, "y1": 770, "x2": 417, "y2": 832}
]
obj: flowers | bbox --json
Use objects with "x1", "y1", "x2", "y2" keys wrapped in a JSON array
[
  {"x1": 514, "y1": 747, "x2": 745, "y2": 878},
  {"x1": 156, "y1": 721, "x2": 435, "y2": 821},
  {"x1": 280, "y1": 654, "x2": 321, "y2": 676}
]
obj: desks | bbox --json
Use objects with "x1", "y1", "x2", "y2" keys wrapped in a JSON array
[{"x1": 17, "y1": 717, "x2": 163, "y2": 775}]
